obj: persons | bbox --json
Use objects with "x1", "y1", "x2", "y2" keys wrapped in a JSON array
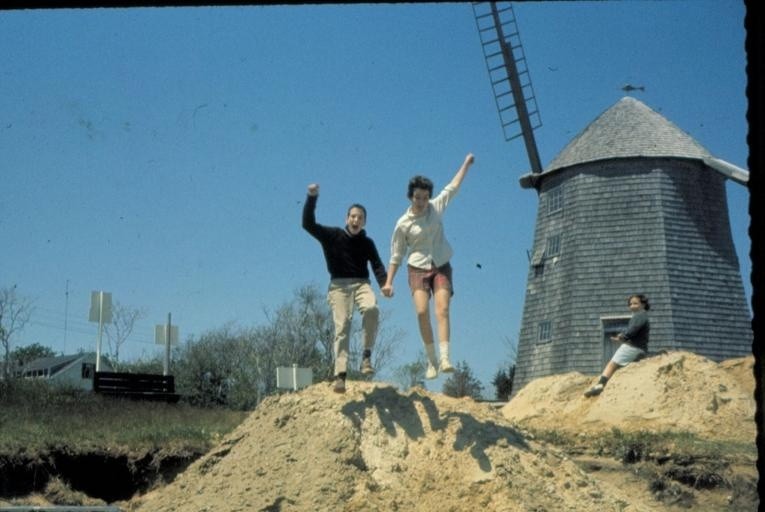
[
  {"x1": 302, "y1": 183, "x2": 387, "y2": 393},
  {"x1": 381, "y1": 152, "x2": 474, "y2": 379},
  {"x1": 584, "y1": 295, "x2": 650, "y2": 398}
]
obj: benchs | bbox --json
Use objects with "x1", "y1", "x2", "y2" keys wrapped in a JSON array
[{"x1": 93, "y1": 371, "x2": 181, "y2": 403}]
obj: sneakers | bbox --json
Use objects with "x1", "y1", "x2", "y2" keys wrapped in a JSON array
[
  {"x1": 439, "y1": 360, "x2": 455, "y2": 373},
  {"x1": 360, "y1": 358, "x2": 374, "y2": 375},
  {"x1": 584, "y1": 384, "x2": 604, "y2": 397},
  {"x1": 426, "y1": 364, "x2": 438, "y2": 379},
  {"x1": 334, "y1": 379, "x2": 346, "y2": 393}
]
